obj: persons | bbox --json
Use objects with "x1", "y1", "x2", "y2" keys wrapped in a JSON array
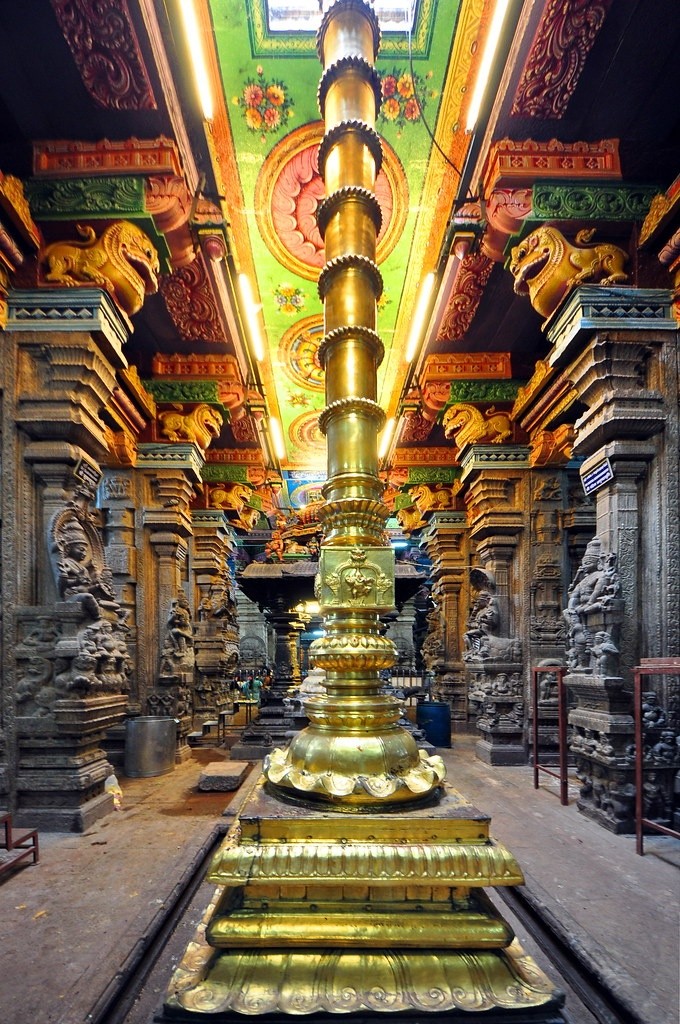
[
  {"x1": 198, "y1": 573, "x2": 240, "y2": 705},
  {"x1": 461, "y1": 568, "x2": 525, "y2": 727},
  {"x1": 539, "y1": 672, "x2": 558, "y2": 700},
  {"x1": 50, "y1": 517, "x2": 131, "y2": 700},
  {"x1": 146, "y1": 588, "x2": 194, "y2": 716},
  {"x1": 265, "y1": 531, "x2": 320, "y2": 562},
  {"x1": 562, "y1": 535, "x2": 680, "y2": 820},
  {"x1": 241, "y1": 676, "x2": 263, "y2": 701},
  {"x1": 420, "y1": 611, "x2": 465, "y2": 720}
]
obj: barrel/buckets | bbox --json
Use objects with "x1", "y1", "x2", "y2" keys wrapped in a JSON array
[
  {"x1": 417, "y1": 702, "x2": 451, "y2": 749},
  {"x1": 125, "y1": 716, "x2": 176, "y2": 778}
]
